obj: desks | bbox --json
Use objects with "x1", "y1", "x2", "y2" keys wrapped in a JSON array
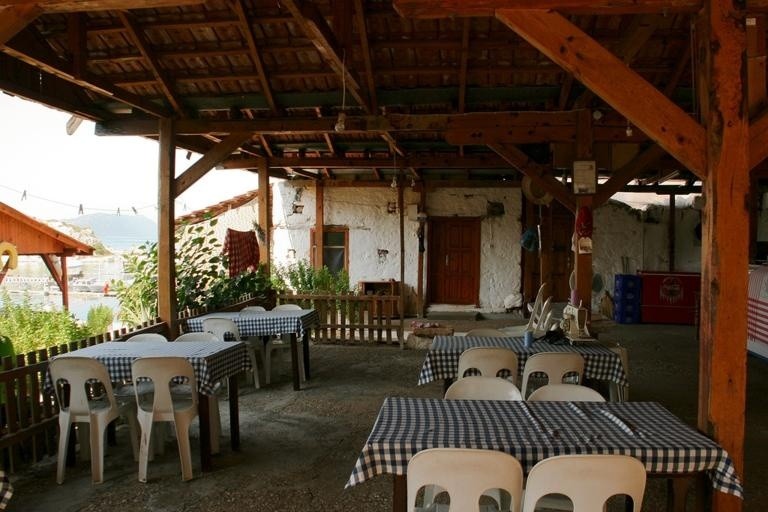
[
  {"x1": 417, "y1": 335, "x2": 628, "y2": 402},
  {"x1": 348, "y1": 400, "x2": 746, "y2": 512}
]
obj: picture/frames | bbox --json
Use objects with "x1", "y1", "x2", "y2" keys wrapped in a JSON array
[{"x1": 570, "y1": 158, "x2": 598, "y2": 198}]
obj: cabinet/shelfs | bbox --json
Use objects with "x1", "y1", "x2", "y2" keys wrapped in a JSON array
[{"x1": 356, "y1": 278, "x2": 402, "y2": 320}]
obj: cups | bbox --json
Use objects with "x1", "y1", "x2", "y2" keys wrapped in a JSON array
[{"x1": 523, "y1": 329, "x2": 533, "y2": 345}]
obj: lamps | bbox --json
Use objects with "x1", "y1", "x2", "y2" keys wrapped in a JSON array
[
  {"x1": 331, "y1": 37, "x2": 347, "y2": 135},
  {"x1": 388, "y1": 145, "x2": 399, "y2": 188}
]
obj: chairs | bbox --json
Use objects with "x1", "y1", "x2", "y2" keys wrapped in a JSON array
[
  {"x1": 405, "y1": 448, "x2": 647, "y2": 511},
  {"x1": 44, "y1": 332, "x2": 249, "y2": 486},
  {"x1": 186, "y1": 303, "x2": 315, "y2": 391},
  {"x1": 443, "y1": 325, "x2": 605, "y2": 402}
]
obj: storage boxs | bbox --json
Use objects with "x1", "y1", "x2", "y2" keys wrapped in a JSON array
[{"x1": 610, "y1": 273, "x2": 643, "y2": 322}]
